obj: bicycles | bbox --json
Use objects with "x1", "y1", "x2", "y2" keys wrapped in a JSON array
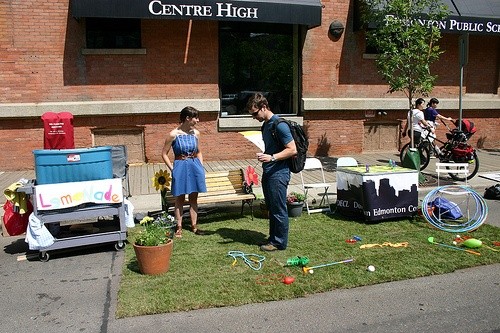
[{"x1": 399, "y1": 126, "x2": 480, "y2": 181}]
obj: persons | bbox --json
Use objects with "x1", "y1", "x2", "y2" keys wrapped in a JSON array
[
  {"x1": 423, "y1": 98, "x2": 453, "y2": 153},
  {"x1": 249, "y1": 94, "x2": 297, "y2": 251},
  {"x1": 162, "y1": 106, "x2": 207, "y2": 237},
  {"x1": 402, "y1": 98, "x2": 435, "y2": 148}
]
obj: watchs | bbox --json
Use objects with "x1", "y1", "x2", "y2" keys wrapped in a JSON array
[{"x1": 271, "y1": 154, "x2": 276, "y2": 161}]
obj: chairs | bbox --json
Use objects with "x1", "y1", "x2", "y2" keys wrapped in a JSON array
[
  {"x1": 336, "y1": 157, "x2": 358, "y2": 167},
  {"x1": 435, "y1": 162, "x2": 474, "y2": 228},
  {"x1": 300, "y1": 158, "x2": 333, "y2": 216}
]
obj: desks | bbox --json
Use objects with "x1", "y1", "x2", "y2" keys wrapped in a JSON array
[{"x1": 334, "y1": 165, "x2": 420, "y2": 225}]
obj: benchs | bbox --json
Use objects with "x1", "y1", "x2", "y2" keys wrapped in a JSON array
[{"x1": 160, "y1": 168, "x2": 257, "y2": 221}]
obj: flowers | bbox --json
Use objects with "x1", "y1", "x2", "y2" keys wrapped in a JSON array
[
  {"x1": 244, "y1": 164, "x2": 259, "y2": 186},
  {"x1": 287, "y1": 191, "x2": 308, "y2": 204},
  {"x1": 151, "y1": 168, "x2": 172, "y2": 192},
  {"x1": 134, "y1": 210, "x2": 177, "y2": 246}
]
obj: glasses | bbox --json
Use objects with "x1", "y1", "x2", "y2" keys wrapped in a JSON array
[{"x1": 252, "y1": 110, "x2": 260, "y2": 116}]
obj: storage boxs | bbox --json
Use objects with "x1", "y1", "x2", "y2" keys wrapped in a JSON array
[{"x1": 31, "y1": 145, "x2": 112, "y2": 186}]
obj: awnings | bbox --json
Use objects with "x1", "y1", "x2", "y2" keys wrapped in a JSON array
[
  {"x1": 353, "y1": 0, "x2": 500, "y2": 34},
  {"x1": 70, "y1": 0, "x2": 321, "y2": 31}
]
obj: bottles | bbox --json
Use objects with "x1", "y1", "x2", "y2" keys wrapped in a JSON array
[
  {"x1": 366, "y1": 164, "x2": 369, "y2": 172},
  {"x1": 389, "y1": 159, "x2": 392, "y2": 167},
  {"x1": 392, "y1": 161, "x2": 396, "y2": 166}
]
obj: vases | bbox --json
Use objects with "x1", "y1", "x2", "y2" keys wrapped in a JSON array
[
  {"x1": 287, "y1": 200, "x2": 305, "y2": 218},
  {"x1": 132, "y1": 238, "x2": 174, "y2": 278},
  {"x1": 259, "y1": 200, "x2": 270, "y2": 219},
  {"x1": 156, "y1": 222, "x2": 177, "y2": 240}
]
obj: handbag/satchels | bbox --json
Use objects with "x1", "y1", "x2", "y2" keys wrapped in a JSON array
[
  {"x1": 483, "y1": 184, "x2": 500, "y2": 199},
  {"x1": 4, "y1": 198, "x2": 33, "y2": 236},
  {"x1": 41, "y1": 112, "x2": 76, "y2": 149}
]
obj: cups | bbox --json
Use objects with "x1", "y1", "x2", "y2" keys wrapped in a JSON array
[{"x1": 256, "y1": 153, "x2": 263, "y2": 161}]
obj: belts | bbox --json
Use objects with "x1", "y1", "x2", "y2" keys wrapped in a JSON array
[{"x1": 177, "y1": 153, "x2": 198, "y2": 160}]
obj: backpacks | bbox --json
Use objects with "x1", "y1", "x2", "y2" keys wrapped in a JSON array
[{"x1": 261, "y1": 118, "x2": 309, "y2": 174}]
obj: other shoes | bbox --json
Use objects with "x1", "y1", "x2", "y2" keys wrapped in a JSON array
[
  {"x1": 176, "y1": 227, "x2": 182, "y2": 234},
  {"x1": 257, "y1": 239, "x2": 277, "y2": 251},
  {"x1": 192, "y1": 225, "x2": 200, "y2": 234}
]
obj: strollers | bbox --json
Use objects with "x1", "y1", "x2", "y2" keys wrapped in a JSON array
[{"x1": 439, "y1": 118, "x2": 478, "y2": 153}]
